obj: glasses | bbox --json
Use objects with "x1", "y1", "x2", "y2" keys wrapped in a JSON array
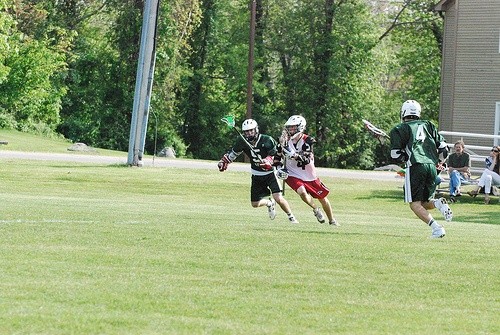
[{"x1": 491, "y1": 149, "x2": 499, "y2": 153}]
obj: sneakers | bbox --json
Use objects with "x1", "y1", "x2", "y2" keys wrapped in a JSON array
[
  {"x1": 432, "y1": 228, "x2": 446, "y2": 239},
  {"x1": 313, "y1": 209, "x2": 324, "y2": 223},
  {"x1": 290, "y1": 217, "x2": 299, "y2": 224},
  {"x1": 267, "y1": 200, "x2": 276, "y2": 220},
  {"x1": 438, "y1": 197, "x2": 452, "y2": 222},
  {"x1": 329, "y1": 219, "x2": 338, "y2": 226}
]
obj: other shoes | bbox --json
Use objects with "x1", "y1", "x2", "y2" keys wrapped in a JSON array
[
  {"x1": 456, "y1": 193, "x2": 462, "y2": 196},
  {"x1": 484, "y1": 197, "x2": 490, "y2": 204},
  {"x1": 450, "y1": 198, "x2": 454, "y2": 203},
  {"x1": 471, "y1": 190, "x2": 478, "y2": 194}
]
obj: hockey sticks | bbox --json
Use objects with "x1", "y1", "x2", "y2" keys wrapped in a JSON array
[
  {"x1": 361, "y1": 118, "x2": 392, "y2": 142},
  {"x1": 221, "y1": 115, "x2": 264, "y2": 164},
  {"x1": 278, "y1": 127, "x2": 291, "y2": 196}
]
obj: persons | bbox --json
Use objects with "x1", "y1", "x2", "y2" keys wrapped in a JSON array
[
  {"x1": 389, "y1": 100, "x2": 453, "y2": 241},
  {"x1": 467, "y1": 146, "x2": 500, "y2": 205},
  {"x1": 436, "y1": 142, "x2": 471, "y2": 203},
  {"x1": 218, "y1": 119, "x2": 299, "y2": 225},
  {"x1": 277, "y1": 115, "x2": 341, "y2": 227}
]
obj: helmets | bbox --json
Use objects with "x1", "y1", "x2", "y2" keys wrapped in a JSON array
[
  {"x1": 284, "y1": 114, "x2": 306, "y2": 139},
  {"x1": 402, "y1": 99, "x2": 421, "y2": 119},
  {"x1": 242, "y1": 120, "x2": 259, "y2": 143}
]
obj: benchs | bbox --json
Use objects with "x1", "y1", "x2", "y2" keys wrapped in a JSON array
[{"x1": 435, "y1": 153, "x2": 500, "y2": 194}]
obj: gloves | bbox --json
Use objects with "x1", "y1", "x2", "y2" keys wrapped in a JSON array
[
  {"x1": 277, "y1": 169, "x2": 289, "y2": 180},
  {"x1": 297, "y1": 158, "x2": 310, "y2": 167},
  {"x1": 217, "y1": 154, "x2": 231, "y2": 172},
  {"x1": 259, "y1": 155, "x2": 273, "y2": 172}
]
